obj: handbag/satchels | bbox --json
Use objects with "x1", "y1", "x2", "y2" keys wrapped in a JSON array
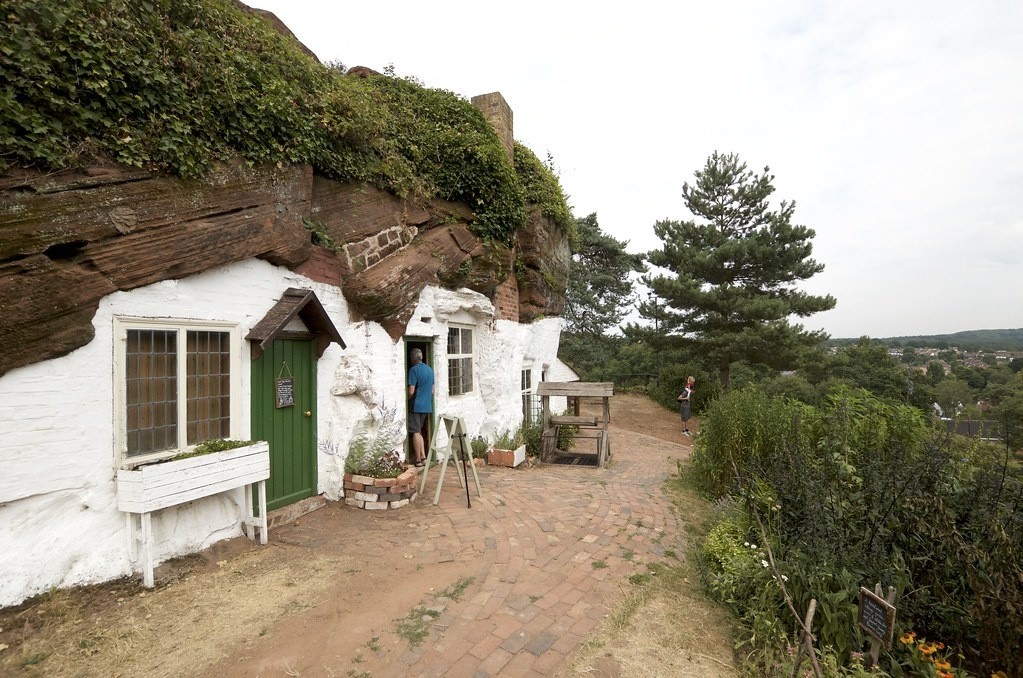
[{"x1": 677, "y1": 390, "x2": 686, "y2": 403}]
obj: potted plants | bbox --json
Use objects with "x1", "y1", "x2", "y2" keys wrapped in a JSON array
[
  {"x1": 117, "y1": 439, "x2": 270, "y2": 589},
  {"x1": 488, "y1": 426, "x2": 525, "y2": 467}
]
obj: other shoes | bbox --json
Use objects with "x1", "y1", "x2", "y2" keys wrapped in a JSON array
[
  {"x1": 681, "y1": 432, "x2": 689, "y2": 437},
  {"x1": 687, "y1": 430, "x2": 693, "y2": 435}
]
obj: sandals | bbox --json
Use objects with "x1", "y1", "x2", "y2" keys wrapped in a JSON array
[
  {"x1": 421, "y1": 458, "x2": 428, "y2": 466},
  {"x1": 411, "y1": 460, "x2": 423, "y2": 467}
]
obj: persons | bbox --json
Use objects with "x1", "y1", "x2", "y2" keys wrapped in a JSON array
[
  {"x1": 678, "y1": 375, "x2": 696, "y2": 436},
  {"x1": 407, "y1": 347, "x2": 434, "y2": 467}
]
